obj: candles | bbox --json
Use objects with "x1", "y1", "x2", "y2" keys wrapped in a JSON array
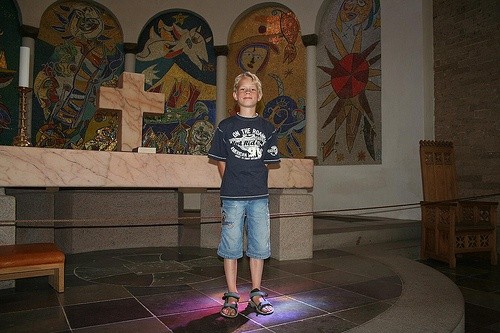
[{"x1": 18, "y1": 44, "x2": 31, "y2": 87}]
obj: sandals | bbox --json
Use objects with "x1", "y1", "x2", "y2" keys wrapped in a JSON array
[
  {"x1": 221, "y1": 292, "x2": 240, "y2": 318},
  {"x1": 248, "y1": 290, "x2": 275, "y2": 315}
]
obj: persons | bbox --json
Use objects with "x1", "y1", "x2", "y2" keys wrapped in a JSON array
[{"x1": 207, "y1": 72, "x2": 281, "y2": 318}]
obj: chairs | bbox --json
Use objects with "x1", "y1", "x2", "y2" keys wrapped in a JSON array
[{"x1": 419, "y1": 139, "x2": 499, "y2": 270}]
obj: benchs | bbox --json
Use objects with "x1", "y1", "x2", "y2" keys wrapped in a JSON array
[{"x1": 0, "y1": 243, "x2": 66, "y2": 292}]
